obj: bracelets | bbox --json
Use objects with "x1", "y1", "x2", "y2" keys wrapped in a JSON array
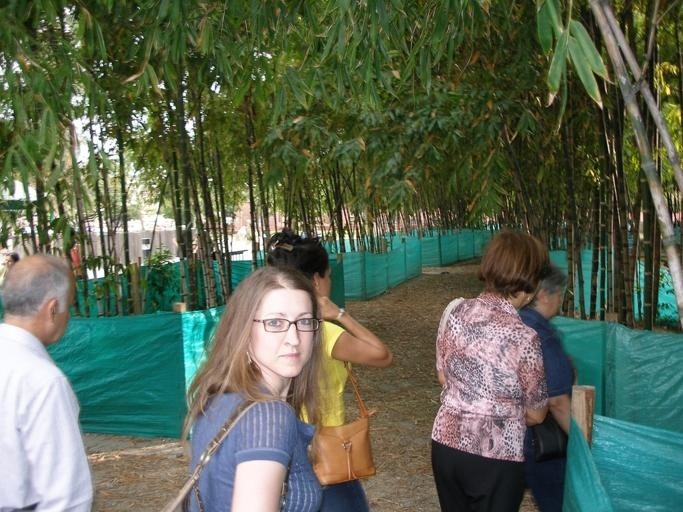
[{"x1": 338, "y1": 307, "x2": 344, "y2": 319}]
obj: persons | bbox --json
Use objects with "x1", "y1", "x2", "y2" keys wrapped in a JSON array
[
  {"x1": 267, "y1": 232, "x2": 392, "y2": 511},
  {"x1": 431, "y1": 230, "x2": 552, "y2": 511},
  {"x1": 518, "y1": 265, "x2": 572, "y2": 511},
  {"x1": 182, "y1": 263, "x2": 321, "y2": 512},
  {"x1": 0, "y1": 238, "x2": 95, "y2": 511}
]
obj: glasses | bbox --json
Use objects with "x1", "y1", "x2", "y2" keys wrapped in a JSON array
[{"x1": 254, "y1": 317, "x2": 320, "y2": 334}]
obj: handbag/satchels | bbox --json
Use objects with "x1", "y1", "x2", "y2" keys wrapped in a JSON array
[
  {"x1": 306, "y1": 415, "x2": 376, "y2": 487},
  {"x1": 532, "y1": 417, "x2": 569, "y2": 464}
]
